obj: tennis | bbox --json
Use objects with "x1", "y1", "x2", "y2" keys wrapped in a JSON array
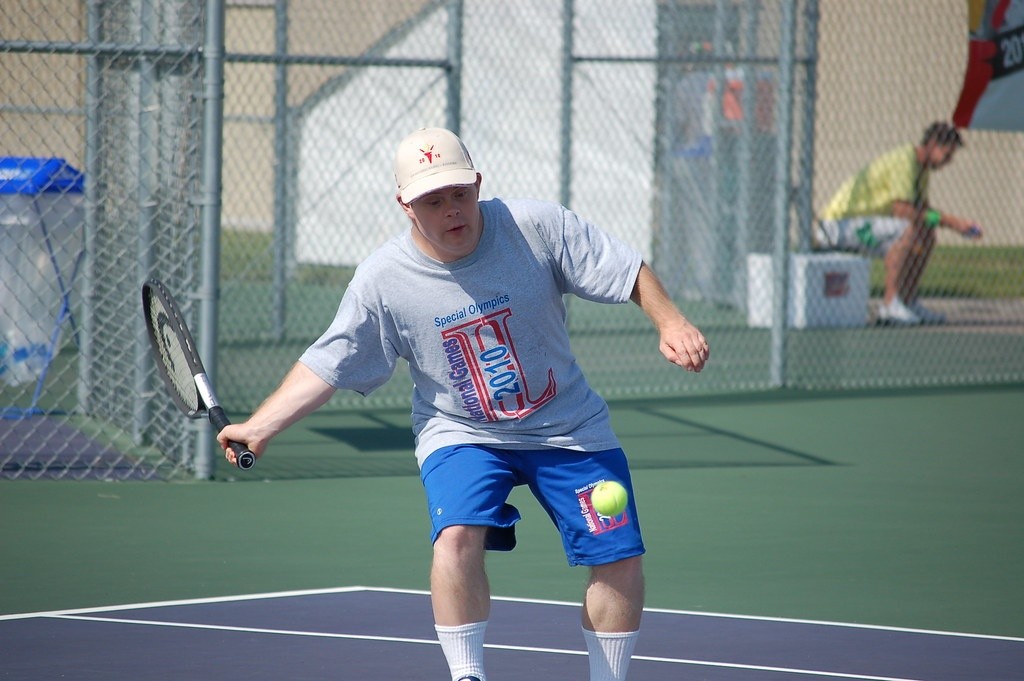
[{"x1": 590, "y1": 480, "x2": 629, "y2": 518}]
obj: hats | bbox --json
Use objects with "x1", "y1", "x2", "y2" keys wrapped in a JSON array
[{"x1": 393, "y1": 128, "x2": 477, "y2": 204}]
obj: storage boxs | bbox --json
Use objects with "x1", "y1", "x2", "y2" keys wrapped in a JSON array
[{"x1": 748, "y1": 249, "x2": 867, "y2": 330}]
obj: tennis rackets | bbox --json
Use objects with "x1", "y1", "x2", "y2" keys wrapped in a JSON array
[{"x1": 141, "y1": 277, "x2": 257, "y2": 472}]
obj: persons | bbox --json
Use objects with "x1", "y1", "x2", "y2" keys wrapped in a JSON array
[
  {"x1": 817, "y1": 120, "x2": 984, "y2": 327},
  {"x1": 214, "y1": 126, "x2": 709, "y2": 681}
]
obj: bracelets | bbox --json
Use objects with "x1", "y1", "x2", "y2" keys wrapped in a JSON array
[{"x1": 923, "y1": 209, "x2": 942, "y2": 227}]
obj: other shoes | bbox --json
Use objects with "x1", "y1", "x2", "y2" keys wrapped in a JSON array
[{"x1": 876, "y1": 297, "x2": 943, "y2": 327}]
